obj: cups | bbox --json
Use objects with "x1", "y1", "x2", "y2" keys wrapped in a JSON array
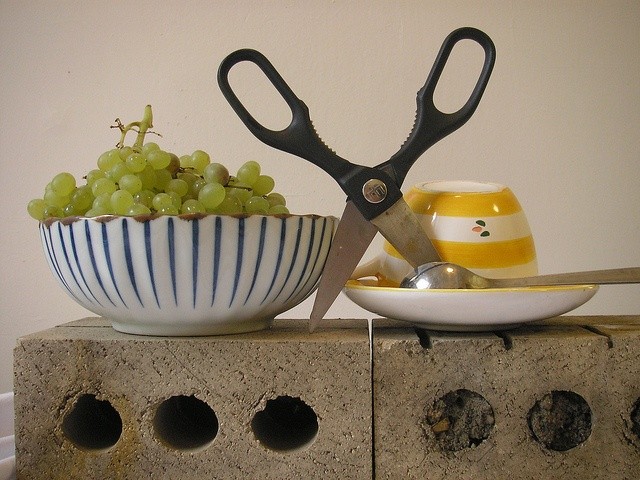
[{"x1": 350, "y1": 181, "x2": 541, "y2": 288}]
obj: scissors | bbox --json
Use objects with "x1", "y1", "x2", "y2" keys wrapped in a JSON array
[{"x1": 217, "y1": 27, "x2": 496, "y2": 333}]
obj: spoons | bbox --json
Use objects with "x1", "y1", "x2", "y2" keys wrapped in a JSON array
[{"x1": 401, "y1": 262, "x2": 640, "y2": 286}]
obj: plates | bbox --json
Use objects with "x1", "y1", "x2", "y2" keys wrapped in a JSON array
[{"x1": 340, "y1": 285, "x2": 600, "y2": 333}]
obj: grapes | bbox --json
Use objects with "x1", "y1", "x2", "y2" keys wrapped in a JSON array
[{"x1": 27, "y1": 103, "x2": 290, "y2": 219}]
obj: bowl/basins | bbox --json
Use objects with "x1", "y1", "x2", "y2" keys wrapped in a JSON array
[{"x1": 39, "y1": 213, "x2": 339, "y2": 337}]
